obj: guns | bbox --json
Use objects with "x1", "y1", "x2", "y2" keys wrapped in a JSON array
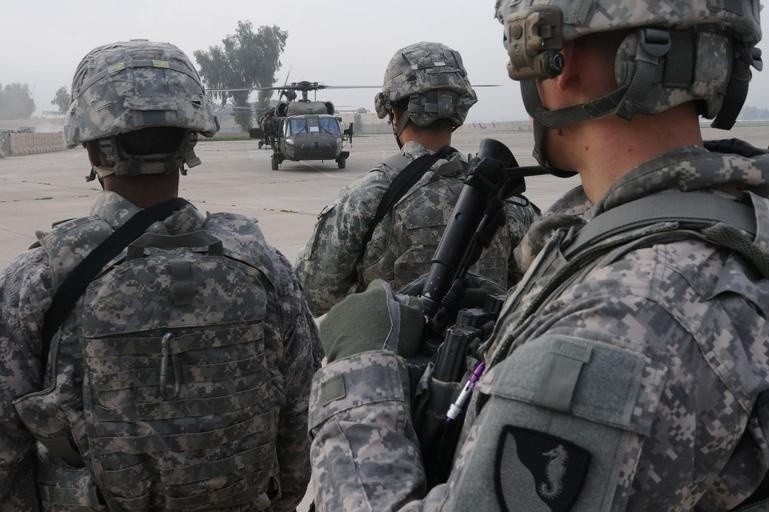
[{"x1": 404, "y1": 138, "x2": 526, "y2": 395}]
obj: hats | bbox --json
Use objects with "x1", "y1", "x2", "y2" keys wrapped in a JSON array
[
  {"x1": 374, "y1": 40, "x2": 478, "y2": 128},
  {"x1": 494, "y1": 1, "x2": 763, "y2": 119},
  {"x1": 64, "y1": 39, "x2": 222, "y2": 150}
]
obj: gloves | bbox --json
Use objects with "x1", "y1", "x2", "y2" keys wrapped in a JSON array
[{"x1": 313, "y1": 279, "x2": 425, "y2": 364}]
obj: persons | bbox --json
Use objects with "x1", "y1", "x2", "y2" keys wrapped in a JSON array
[
  {"x1": 290, "y1": 42, "x2": 543, "y2": 330},
  {"x1": 312, "y1": 0, "x2": 768, "y2": 512},
  {"x1": 3, "y1": 40, "x2": 323, "y2": 512}
]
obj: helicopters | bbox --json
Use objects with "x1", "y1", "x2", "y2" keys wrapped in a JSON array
[{"x1": 204, "y1": 80, "x2": 503, "y2": 170}]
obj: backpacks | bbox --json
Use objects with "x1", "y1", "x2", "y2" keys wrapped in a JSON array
[
  {"x1": 36, "y1": 213, "x2": 288, "y2": 512},
  {"x1": 362, "y1": 154, "x2": 538, "y2": 299}
]
